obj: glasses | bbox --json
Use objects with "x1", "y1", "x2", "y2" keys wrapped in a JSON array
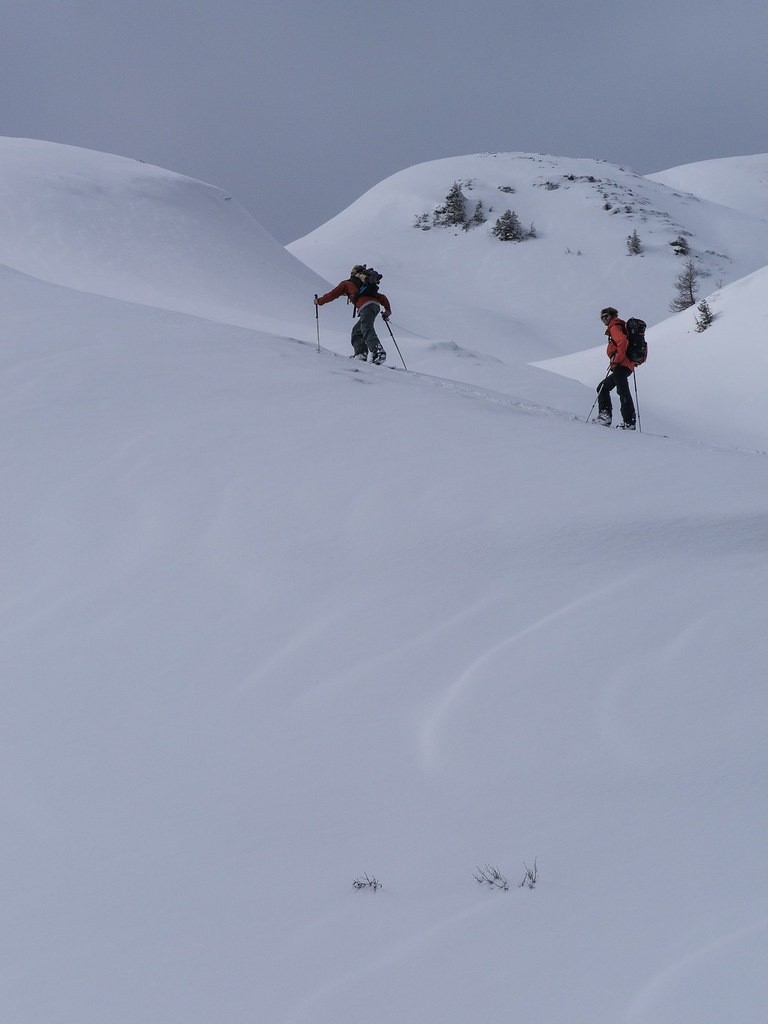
[{"x1": 601, "y1": 314, "x2": 609, "y2": 320}]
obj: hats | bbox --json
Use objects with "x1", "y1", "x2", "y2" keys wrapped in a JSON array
[
  {"x1": 602, "y1": 307, "x2": 619, "y2": 318},
  {"x1": 350, "y1": 265, "x2": 365, "y2": 274}
]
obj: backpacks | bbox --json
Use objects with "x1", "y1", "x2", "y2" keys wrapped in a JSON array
[
  {"x1": 345, "y1": 267, "x2": 382, "y2": 305},
  {"x1": 608, "y1": 317, "x2": 647, "y2": 367}
]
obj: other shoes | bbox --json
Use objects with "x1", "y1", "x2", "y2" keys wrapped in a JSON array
[
  {"x1": 371, "y1": 352, "x2": 386, "y2": 365},
  {"x1": 616, "y1": 421, "x2": 636, "y2": 430},
  {"x1": 350, "y1": 353, "x2": 367, "y2": 361},
  {"x1": 592, "y1": 410, "x2": 612, "y2": 426}
]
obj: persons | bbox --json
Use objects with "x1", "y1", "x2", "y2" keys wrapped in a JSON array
[
  {"x1": 313, "y1": 279, "x2": 392, "y2": 365},
  {"x1": 592, "y1": 306, "x2": 637, "y2": 431}
]
